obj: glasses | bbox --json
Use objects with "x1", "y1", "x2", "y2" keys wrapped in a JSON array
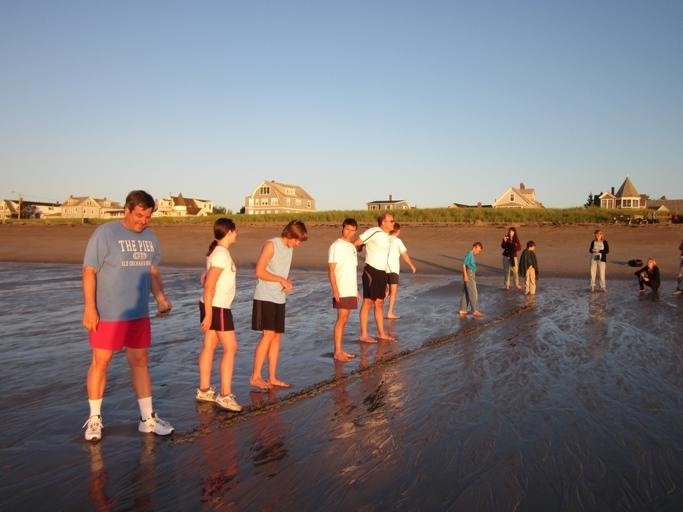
[{"x1": 386, "y1": 220, "x2": 395, "y2": 223}]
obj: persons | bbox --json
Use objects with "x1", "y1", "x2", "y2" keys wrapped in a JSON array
[
  {"x1": 353, "y1": 212, "x2": 396, "y2": 343},
  {"x1": 672, "y1": 239, "x2": 683, "y2": 295},
  {"x1": 519, "y1": 241, "x2": 539, "y2": 295},
  {"x1": 195, "y1": 218, "x2": 243, "y2": 412},
  {"x1": 249, "y1": 219, "x2": 309, "y2": 389},
  {"x1": 82, "y1": 190, "x2": 175, "y2": 441},
  {"x1": 589, "y1": 230, "x2": 610, "y2": 293},
  {"x1": 501, "y1": 227, "x2": 522, "y2": 290},
  {"x1": 328, "y1": 218, "x2": 358, "y2": 362},
  {"x1": 634, "y1": 257, "x2": 661, "y2": 295},
  {"x1": 459, "y1": 242, "x2": 485, "y2": 317},
  {"x1": 384, "y1": 222, "x2": 416, "y2": 319}
]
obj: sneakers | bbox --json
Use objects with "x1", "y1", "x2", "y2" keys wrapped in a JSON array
[
  {"x1": 82, "y1": 415, "x2": 104, "y2": 441},
  {"x1": 138, "y1": 412, "x2": 175, "y2": 436},
  {"x1": 195, "y1": 387, "x2": 217, "y2": 402},
  {"x1": 215, "y1": 394, "x2": 242, "y2": 412}
]
